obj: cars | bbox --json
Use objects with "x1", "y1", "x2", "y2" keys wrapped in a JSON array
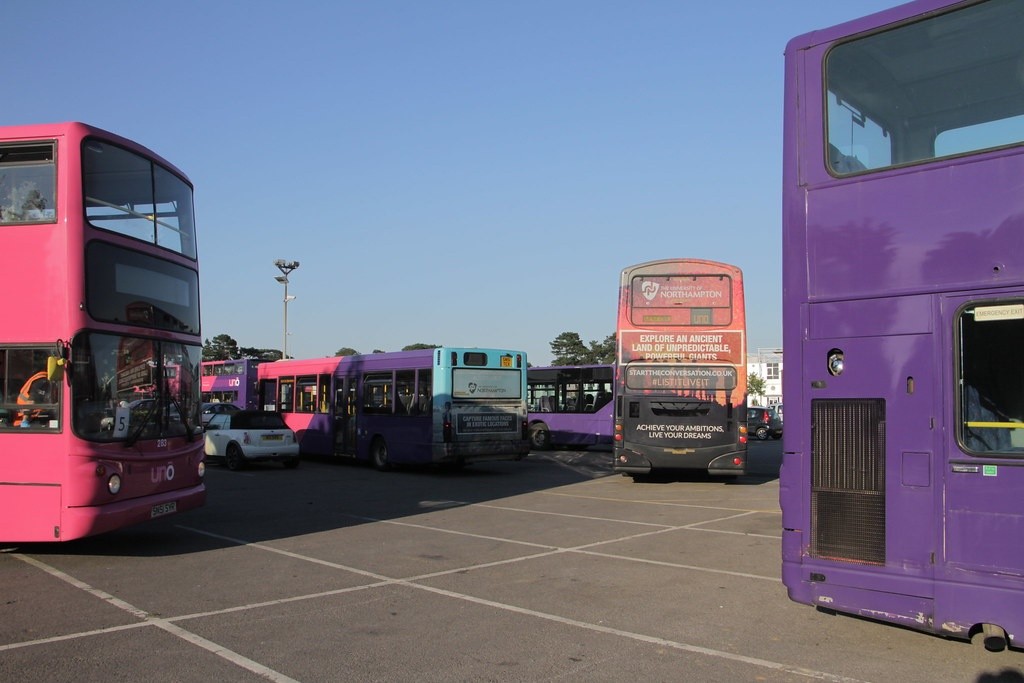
[
  {"x1": 0, "y1": 121, "x2": 207, "y2": 544},
  {"x1": 162, "y1": 400, "x2": 204, "y2": 423},
  {"x1": 201, "y1": 403, "x2": 241, "y2": 414},
  {"x1": 767, "y1": 404, "x2": 783, "y2": 430},
  {"x1": 744, "y1": 407, "x2": 782, "y2": 441},
  {"x1": 126, "y1": 399, "x2": 158, "y2": 415},
  {"x1": 201, "y1": 409, "x2": 301, "y2": 471}
]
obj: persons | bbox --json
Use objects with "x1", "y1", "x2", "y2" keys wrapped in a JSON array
[
  {"x1": 443, "y1": 402, "x2": 455, "y2": 456},
  {"x1": 13, "y1": 370, "x2": 58, "y2": 426}
]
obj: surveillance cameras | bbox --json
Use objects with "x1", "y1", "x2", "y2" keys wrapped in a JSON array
[{"x1": 288, "y1": 296, "x2": 296, "y2": 300}]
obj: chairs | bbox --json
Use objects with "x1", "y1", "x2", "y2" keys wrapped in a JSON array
[
  {"x1": 539, "y1": 392, "x2": 613, "y2": 414},
  {"x1": 364, "y1": 393, "x2": 433, "y2": 415},
  {"x1": 16, "y1": 371, "x2": 50, "y2": 421}
]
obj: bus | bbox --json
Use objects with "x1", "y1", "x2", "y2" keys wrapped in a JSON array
[
  {"x1": 521, "y1": 363, "x2": 614, "y2": 450},
  {"x1": 779, "y1": 0, "x2": 1024, "y2": 661},
  {"x1": 134, "y1": 358, "x2": 278, "y2": 414},
  {"x1": 256, "y1": 346, "x2": 527, "y2": 471},
  {"x1": 614, "y1": 256, "x2": 750, "y2": 477},
  {"x1": 74, "y1": 453, "x2": 78, "y2": 457}
]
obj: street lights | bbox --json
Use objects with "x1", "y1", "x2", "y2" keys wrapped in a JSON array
[{"x1": 272, "y1": 258, "x2": 301, "y2": 356}]
